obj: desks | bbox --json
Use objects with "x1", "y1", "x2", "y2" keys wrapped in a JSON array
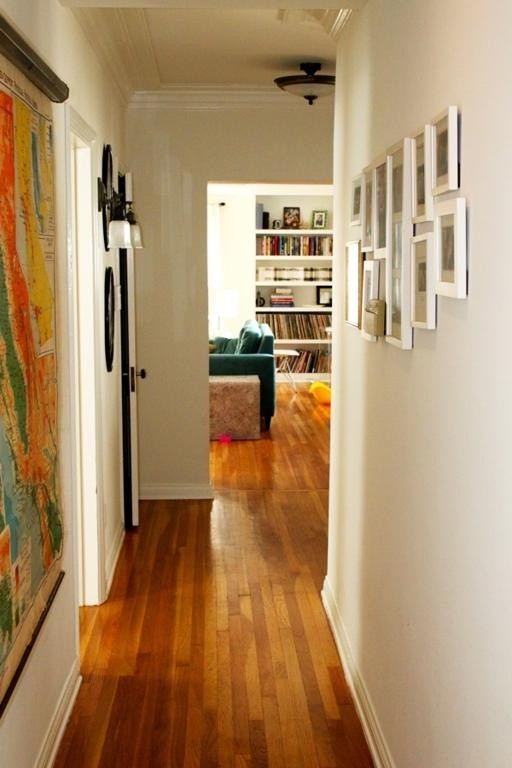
[{"x1": 273, "y1": 349, "x2": 301, "y2": 393}]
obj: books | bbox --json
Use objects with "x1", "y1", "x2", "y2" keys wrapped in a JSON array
[{"x1": 256, "y1": 201, "x2": 334, "y2": 373}]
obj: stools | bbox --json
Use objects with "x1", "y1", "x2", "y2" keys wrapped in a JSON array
[{"x1": 210, "y1": 375, "x2": 261, "y2": 440}]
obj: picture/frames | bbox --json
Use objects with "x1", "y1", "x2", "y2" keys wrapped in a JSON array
[
  {"x1": 311, "y1": 209, "x2": 328, "y2": 230},
  {"x1": 338, "y1": 103, "x2": 471, "y2": 356},
  {"x1": 105, "y1": 265, "x2": 116, "y2": 371},
  {"x1": 282, "y1": 205, "x2": 300, "y2": 229},
  {"x1": 316, "y1": 286, "x2": 332, "y2": 305},
  {"x1": 103, "y1": 144, "x2": 114, "y2": 252}
]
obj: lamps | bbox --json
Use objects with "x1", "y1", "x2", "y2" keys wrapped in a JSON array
[
  {"x1": 98, "y1": 177, "x2": 145, "y2": 249},
  {"x1": 274, "y1": 61, "x2": 334, "y2": 106}
]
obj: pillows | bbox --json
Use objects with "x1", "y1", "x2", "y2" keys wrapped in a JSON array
[{"x1": 214, "y1": 335, "x2": 237, "y2": 355}]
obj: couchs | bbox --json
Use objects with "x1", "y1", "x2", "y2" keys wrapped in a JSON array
[{"x1": 209, "y1": 317, "x2": 275, "y2": 441}]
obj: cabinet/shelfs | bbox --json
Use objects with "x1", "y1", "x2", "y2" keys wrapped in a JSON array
[{"x1": 250, "y1": 192, "x2": 333, "y2": 383}]
